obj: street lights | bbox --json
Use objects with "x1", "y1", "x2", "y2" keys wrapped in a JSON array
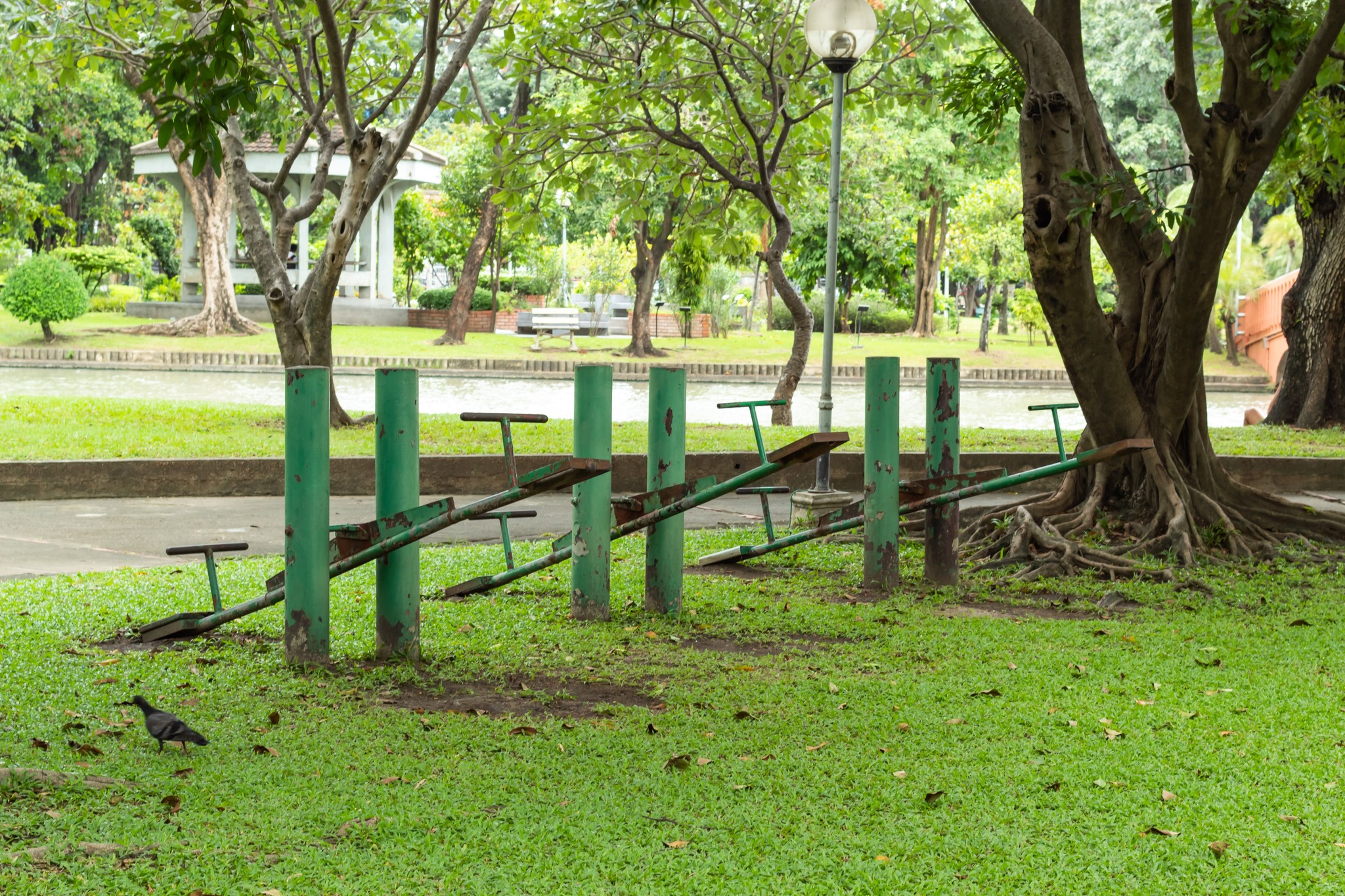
[{"x1": 803, "y1": 0, "x2": 880, "y2": 493}]
